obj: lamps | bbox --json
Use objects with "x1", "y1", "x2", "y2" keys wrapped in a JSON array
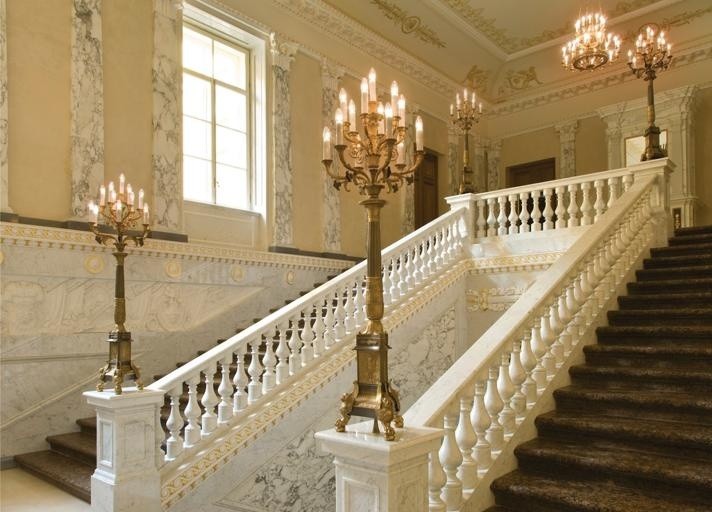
[
  {"x1": 448, "y1": 88, "x2": 483, "y2": 195},
  {"x1": 625, "y1": 25, "x2": 675, "y2": 157},
  {"x1": 321, "y1": 66, "x2": 426, "y2": 442},
  {"x1": 87, "y1": 173, "x2": 152, "y2": 394},
  {"x1": 562, "y1": 0, "x2": 621, "y2": 73}
]
